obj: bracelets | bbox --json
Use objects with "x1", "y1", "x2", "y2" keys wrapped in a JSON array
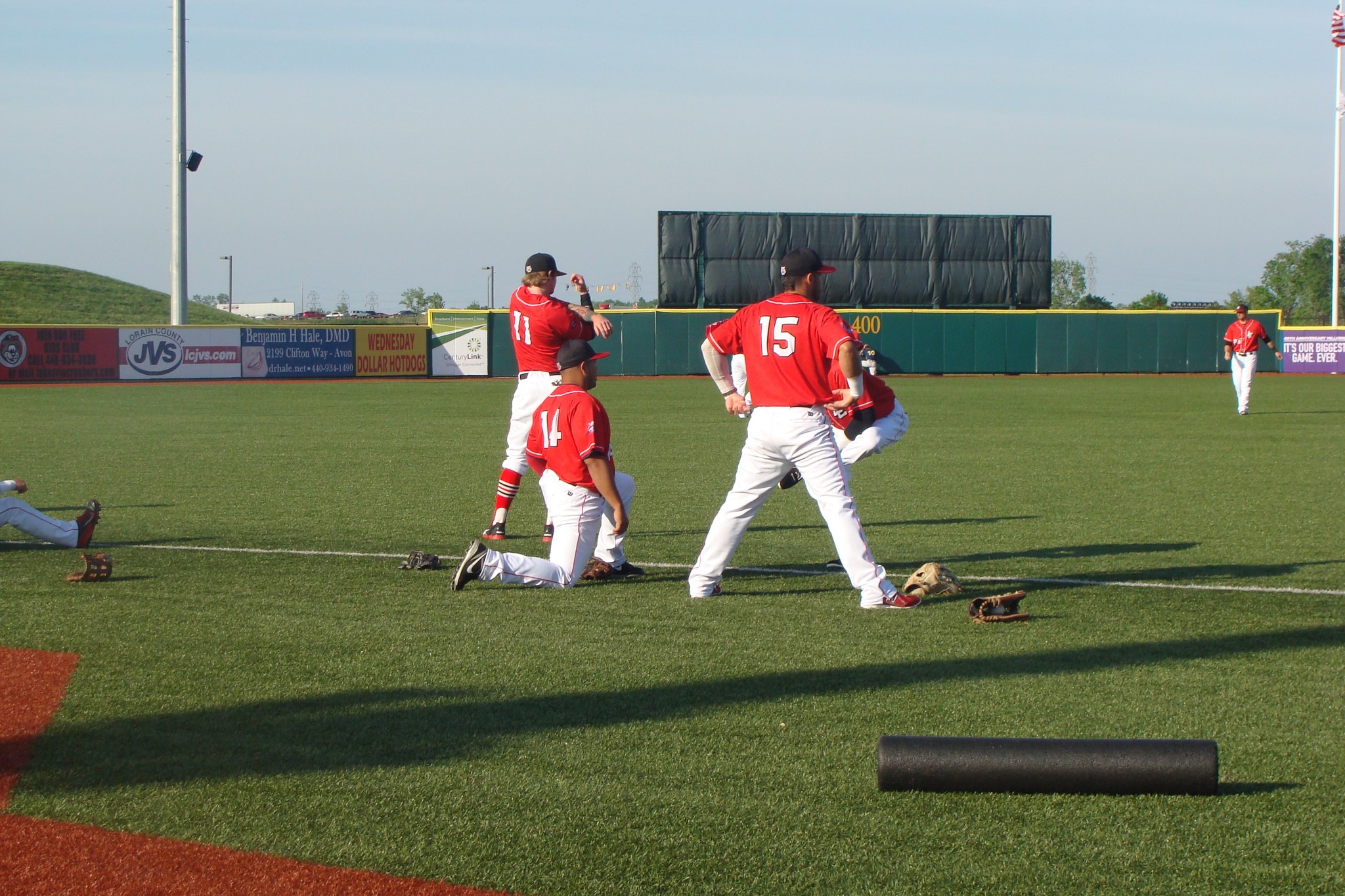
[
  {"x1": 722, "y1": 387, "x2": 737, "y2": 396},
  {"x1": 846, "y1": 372, "x2": 864, "y2": 399}
]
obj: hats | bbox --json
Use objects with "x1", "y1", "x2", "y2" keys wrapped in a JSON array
[
  {"x1": 1233, "y1": 304, "x2": 1248, "y2": 312},
  {"x1": 525, "y1": 253, "x2": 567, "y2": 276},
  {"x1": 781, "y1": 247, "x2": 836, "y2": 276},
  {"x1": 556, "y1": 339, "x2": 610, "y2": 371}
]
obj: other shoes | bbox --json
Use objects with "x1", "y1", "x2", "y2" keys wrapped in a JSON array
[{"x1": 1240, "y1": 411, "x2": 1246, "y2": 415}]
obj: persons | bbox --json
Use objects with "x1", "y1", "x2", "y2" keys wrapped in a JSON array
[
  {"x1": 1271, "y1": 345, "x2": 1278, "y2": 352},
  {"x1": 0, "y1": 479, "x2": 101, "y2": 548},
  {"x1": 859, "y1": 343, "x2": 877, "y2": 376},
  {"x1": 1223, "y1": 304, "x2": 1283, "y2": 415},
  {"x1": 688, "y1": 248, "x2": 920, "y2": 607},
  {"x1": 483, "y1": 252, "x2": 613, "y2": 542},
  {"x1": 242, "y1": 346, "x2": 268, "y2": 378},
  {"x1": 450, "y1": 339, "x2": 644, "y2": 591}
]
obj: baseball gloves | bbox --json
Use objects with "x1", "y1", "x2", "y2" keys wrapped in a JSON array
[
  {"x1": 399, "y1": 550, "x2": 442, "y2": 569},
  {"x1": 968, "y1": 590, "x2": 1030, "y2": 624},
  {"x1": 67, "y1": 552, "x2": 112, "y2": 581},
  {"x1": 902, "y1": 562, "x2": 965, "y2": 595},
  {"x1": 579, "y1": 556, "x2": 616, "y2": 580}
]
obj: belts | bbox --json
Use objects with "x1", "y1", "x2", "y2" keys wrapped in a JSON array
[
  {"x1": 520, "y1": 372, "x2": 561, "y2": 380},
  {"x1": 1239, "y1": 352, "x2": 1253, "y2": 356}
]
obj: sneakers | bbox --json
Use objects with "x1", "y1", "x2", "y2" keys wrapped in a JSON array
[
  {"x1": 542, "y1": 524, "x2": 554, "y2": 542},
  {"x1": 77, "y1": 499, "x2": 101, "y2": 548},
  {"x1": 861, "y1": 591, "x2": 921, "y2": 609},
  {"x1": 611, "y1": 560, "x2": 644, "y2": 576},
  {"x1": 482, "y1": 523, "x2": 505, "y2": 540},
  {"x1": 778, "y1": 465, "x2": 802, "y2": 489},
  {"x1": 450, "y1": 539, "x2": 487, "y2": 592},
  {"x1": 710, "y1": 585, "x2": 722, "y2": 596}
]
